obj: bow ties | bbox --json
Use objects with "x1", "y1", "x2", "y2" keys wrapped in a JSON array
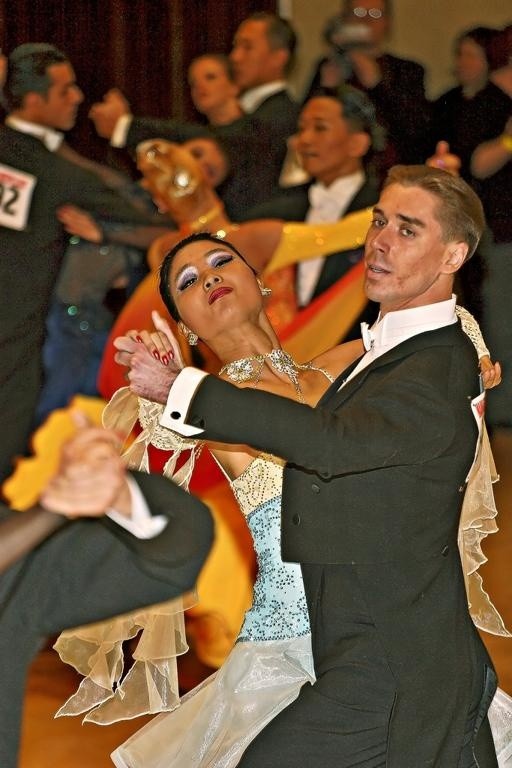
[
  {"x1": 357, "y1": 320, "x2": 382, "y2": 351},
  {"x1": 306, "y1": 183, "x2": 341, "y2": 211}
]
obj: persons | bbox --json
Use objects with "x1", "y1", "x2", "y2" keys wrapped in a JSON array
[
  {"x1": 0, "y1": 40, "x2": 461, "y2": 768},
  {"x1": 114, "y1": 164, "x2": 499, "y2": 768},
  {"x1": 94, "y1": 0, "x2": 510, "y2": 435},
  {"x1": 53, "y1": 228, "x2": 511, "y2": 768}
]
